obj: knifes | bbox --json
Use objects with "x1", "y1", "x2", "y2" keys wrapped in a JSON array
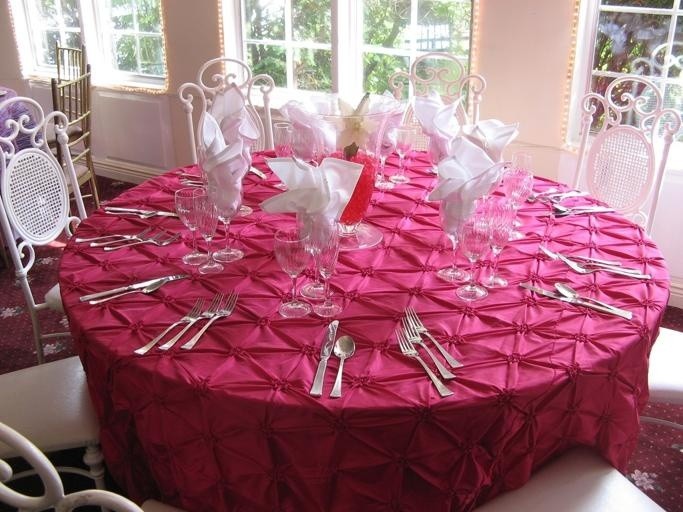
[
  {"x1": 308, "y1": 320, "x2": 339, "y2": 397},
  {"x1": 518, "y1": 283, "x2": 633, "y2": 322},
  {"x1": 104, "y1": 205, "x2": 178, "y2": 219}
]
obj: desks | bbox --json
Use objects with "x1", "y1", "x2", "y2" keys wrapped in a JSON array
[{"x1": 60, "y1": 146, "x2": 667, "y2": 511}]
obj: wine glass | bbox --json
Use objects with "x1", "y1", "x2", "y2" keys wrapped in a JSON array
[
  {"x1": 273, "y1": 209, "x2": 344, "y2": 316},
  {"x1": 435, "y1": 171, "x2": 532, "y2": 302},
  {"x1": 311, "y1": 100, "x2": 417, "y2": 252},
  {"x1": 176, "y1": 186, "x2": 244, "y2": 275}
]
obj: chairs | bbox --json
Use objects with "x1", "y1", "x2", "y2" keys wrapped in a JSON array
[
  {"x1": 570, "y1": 75, "x2": 676, "y2": 238},
  {"x1": 179, "y1": 58, "x2": 275, "y2": 165},
  {"x1": 470, "y1": 448, "x2": 669, "y2": 510},
  {"x1": 387, "y1": 53, "x2": 485, "y2": 151},
  {"x1": 3, "y1": 423, "x2": 187, "y2": 511},
  {"x1": 1, "y1": 355, "x2": 106, "y2": 512},
  {"x1": 51, "y1": 65, "x2": 101, "y2": 235},
  {"x1": 43, "y1": 45, "x2": 99, "y2": 198}
]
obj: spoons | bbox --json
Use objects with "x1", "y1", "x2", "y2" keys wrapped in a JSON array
[
  {"x1": 330, "y1": 336, "x2": 356, "y2": 398},
  {"x1": 553, "y1": 203, "x2": 605, "y2": 212},
  {"x1": 90, "y1": 277, "x2": 169, "y2": 305},
  {"x1": 554, "y1": 281, "x2": 633, "y2": 317}
]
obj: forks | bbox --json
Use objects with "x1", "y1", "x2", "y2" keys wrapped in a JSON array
[
  {"x1": 393, "y1": 307, "x2": 464, "y2": 398},
  {"x1": 105, "y1": 209, "x2": 158, "y2": 220},
  {"x1": 537, "y1": 244, "x2": 653, "y2": 281},
  {"x1": 134, "y1": 291, "x2": 239, "y2": 357},
  {"x1": 75, "y1": 227, "x2": 181, "y2": 252},
  {"x1": 545, "y1": 191, "x2": 591, "y2": 204}
]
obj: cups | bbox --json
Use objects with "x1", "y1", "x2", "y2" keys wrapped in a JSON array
[
  {"x1": 272, "y1": 123, "x2": 293, "y2": 157},
  {"x1": 512, "y1": 153, "x2": 533, "y2": 171}
]
